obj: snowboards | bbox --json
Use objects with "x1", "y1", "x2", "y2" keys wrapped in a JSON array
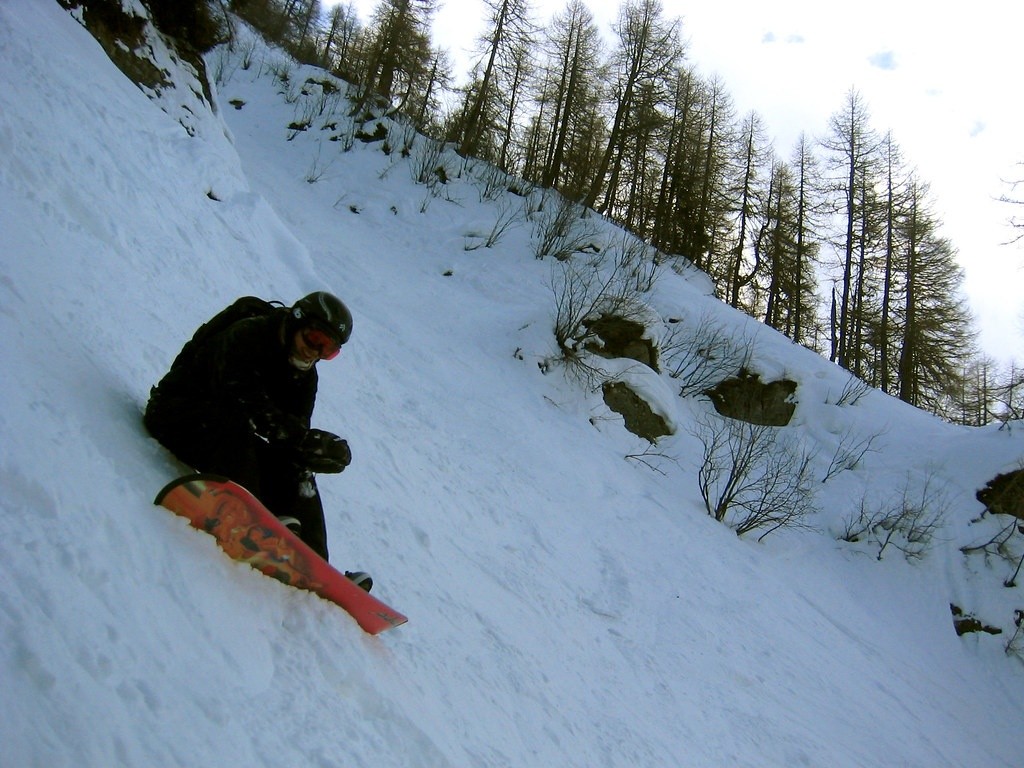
[{"x1": 151, "y1": 472, "x2": 410, "y2": 640}]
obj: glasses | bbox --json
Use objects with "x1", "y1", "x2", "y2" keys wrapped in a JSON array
[{"x1": 301, "y1": 322, "x2": 342, "y2": 360}]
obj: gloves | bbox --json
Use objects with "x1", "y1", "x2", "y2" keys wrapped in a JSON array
[{"x1": 294, "y1": 428, "x2": 351, "y2": 474}]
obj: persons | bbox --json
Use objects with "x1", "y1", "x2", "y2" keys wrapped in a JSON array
[{"x1": 143, "y1": 291, "x2": 373, "y2": 593}]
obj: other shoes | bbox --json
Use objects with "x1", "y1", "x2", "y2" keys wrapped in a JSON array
[
  {"x1": 344, "y1": 571, "x2": 373, "y2": 593},
  {"x1": 278, "y1": 515, "x2": 300, "y2": 535}
]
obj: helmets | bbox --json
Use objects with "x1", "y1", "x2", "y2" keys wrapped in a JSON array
[{"x1": 291, "y1": 291, "x2": 352, "y2": 344}]
